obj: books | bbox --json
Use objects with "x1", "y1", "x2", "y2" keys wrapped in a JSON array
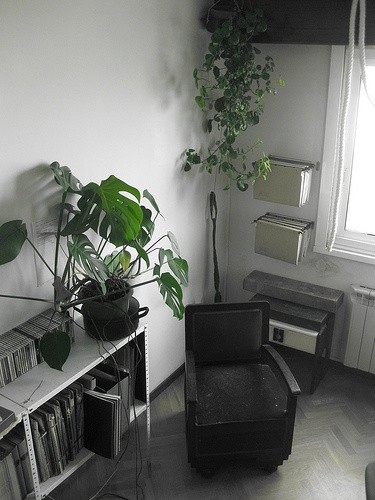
[{"x1": 81, "y1": 387, "x2": 121, "y2": 460}]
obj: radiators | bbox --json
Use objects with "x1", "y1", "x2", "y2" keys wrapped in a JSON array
[{"x1": 340, "y1": 284, "x2": 375, "y2": 375}]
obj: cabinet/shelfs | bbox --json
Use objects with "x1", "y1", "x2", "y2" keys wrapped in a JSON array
[
  {"x1": 231, "y1": 293, "x2": 335, "y2": 394},
  {"x1": 0, "y1": 315, "x2": 150, "y2": 500}
]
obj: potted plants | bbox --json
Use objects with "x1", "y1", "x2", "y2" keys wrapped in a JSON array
[{"x1": 0, "y1": 161, "x2": 189, "y2": 372}]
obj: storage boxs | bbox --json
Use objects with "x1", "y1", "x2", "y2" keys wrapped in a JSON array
[{"x1": 243, "y1": 270, "x2": 344, "y2": 313}]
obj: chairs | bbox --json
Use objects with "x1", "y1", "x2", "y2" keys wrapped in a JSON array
[{"x1": 185, "y1": 302, "x2": 301, "y2": 471}]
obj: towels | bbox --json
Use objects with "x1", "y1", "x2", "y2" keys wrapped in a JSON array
[
  {"x1": 253, "y1": 159, "x2": 313, "y2": 207},
  {"x1": 254, "y1": 217, "x2": 311, "y2": 266}
]
